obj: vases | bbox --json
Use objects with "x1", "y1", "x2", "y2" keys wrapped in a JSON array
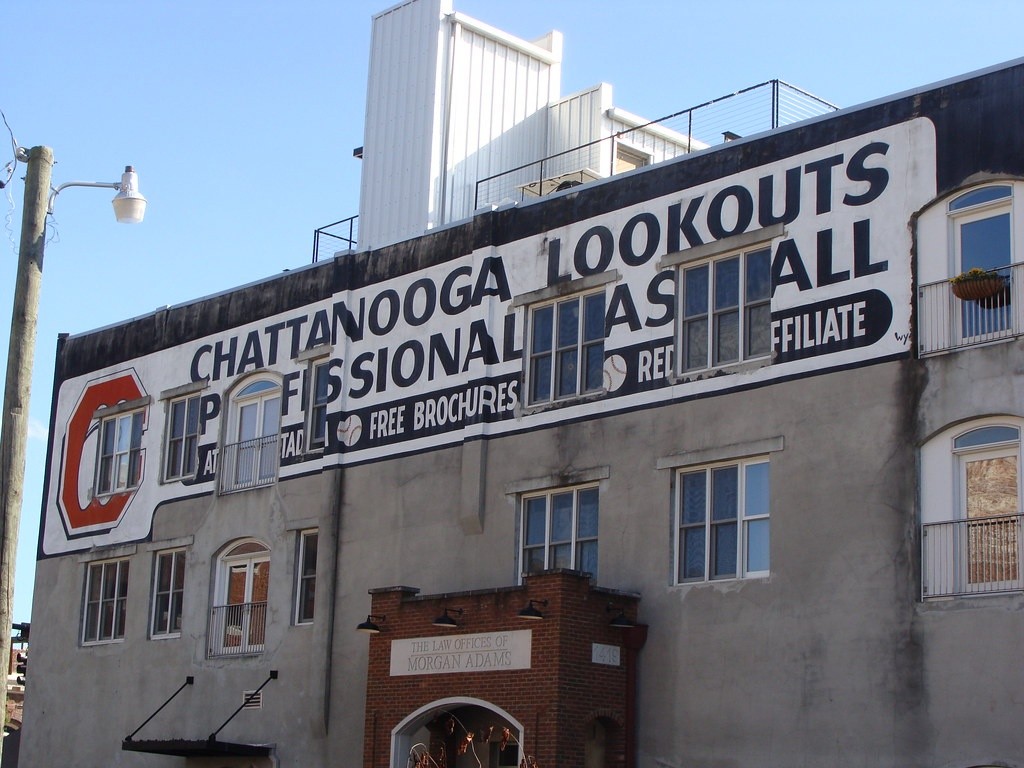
[{"x1": 951, "y1": 278, "x2": 1004, "y2": 301}]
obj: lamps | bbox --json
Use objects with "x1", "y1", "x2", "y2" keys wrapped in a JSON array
[
  {"x1": 356, "y1": 614, "x2": 387, "y2": 634},
  {"x1": 517, "y1": 600, "x2": 548, "y2": 619},
  {"x1": 606, "y1": 606, "x2": 635, "y2": 627},
  {"x1": 432, "y1": 607, "x2": 463, "y2": 627}
]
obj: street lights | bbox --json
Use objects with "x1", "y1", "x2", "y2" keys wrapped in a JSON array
[{"x1": 0, "y1": 146, "x2": 145, "y2": 737}]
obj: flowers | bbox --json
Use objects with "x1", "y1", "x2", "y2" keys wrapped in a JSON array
[{"x1": 949, "y1": 269, "x2": 998, "y2": 285}]
